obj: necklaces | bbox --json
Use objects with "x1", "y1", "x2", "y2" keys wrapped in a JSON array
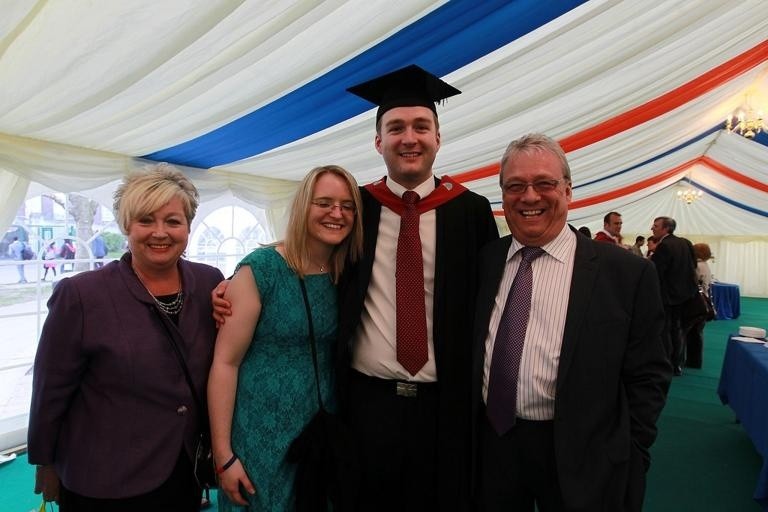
[
  {"x1": 304, "y1": 250, "x2": 331, "y2": 274},
  {"x1": 128, "y1": 267, "x2": 185, "y2": 314}
]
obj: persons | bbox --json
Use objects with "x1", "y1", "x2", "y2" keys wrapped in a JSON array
[
  {"x1": 203, "y1": 106, "x2": 502, "y2": 510},
  {"x1": 40, "y1": 240, "x2": 57, "y2": 281},
  {"x1": 571, "y1": 209, "x2": 713, "y2": 379},
  {"x1": 91, "y1": 230, "x2": 108, "y2": 268},
  {"x1": 207, "y1": 165, "x2": 366, "y2": 512},
  {"x1": 478, "y1": 133, "x2": 675, "y2": 510},
  {"x1": 25, "y1": 159, "x2": 230, "y2": 512},
  {"x1": 58, "y1": 237, "x2": 76, "y2": 274},
  {"x1": 7, "y1": 236, "x2": 28, "y2": 284}
]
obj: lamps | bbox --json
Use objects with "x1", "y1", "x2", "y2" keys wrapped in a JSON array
[
  {"x1": 724, "y1": 72, "x2": 768, "y2": 138},
  {"x1": 675, "y1": 173, "x2": 704, "y2": 203}
]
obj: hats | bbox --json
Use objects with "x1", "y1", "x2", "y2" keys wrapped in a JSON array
[{"x1": 347, "y1": 64, "x2": 461, "y2": 125}]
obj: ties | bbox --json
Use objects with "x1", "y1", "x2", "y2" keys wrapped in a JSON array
[
  {"x1": 486, "y1": 246, "x2": 545, "y2": 436},
  {"x1": 396, "y1": 191, "x2": 428, "y2": 376}
]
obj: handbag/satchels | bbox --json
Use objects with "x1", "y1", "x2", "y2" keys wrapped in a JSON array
[
  {"x1": 193, "y1": 433, "x2": 220, "y2": 490},
  {"x1": 21, "y1": 243, "x2": 32, "y2": 260},
  {"x1": 700, "y1": 292, "x2": 715, "y2": 321}
]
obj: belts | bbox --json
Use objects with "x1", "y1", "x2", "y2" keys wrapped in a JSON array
[{"x1": 352, "y1": 371, "x2": 439, "y2": 398}]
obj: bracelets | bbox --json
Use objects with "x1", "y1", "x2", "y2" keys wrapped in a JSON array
[{"x1": 213, "y1": 454, "x2": 237, "y2": 477}]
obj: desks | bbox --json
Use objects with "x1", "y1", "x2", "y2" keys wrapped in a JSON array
[
  {"x1": 712, "y1": 282, "x2": 738, "y2": 318},
  {"x1": 719, "y1": 331, "x2": 768, "y2": 457}
]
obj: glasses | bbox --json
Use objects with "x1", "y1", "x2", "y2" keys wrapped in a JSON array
[{"x1": 312, "y1": 202, "x2": 357, "y2": 214}]
